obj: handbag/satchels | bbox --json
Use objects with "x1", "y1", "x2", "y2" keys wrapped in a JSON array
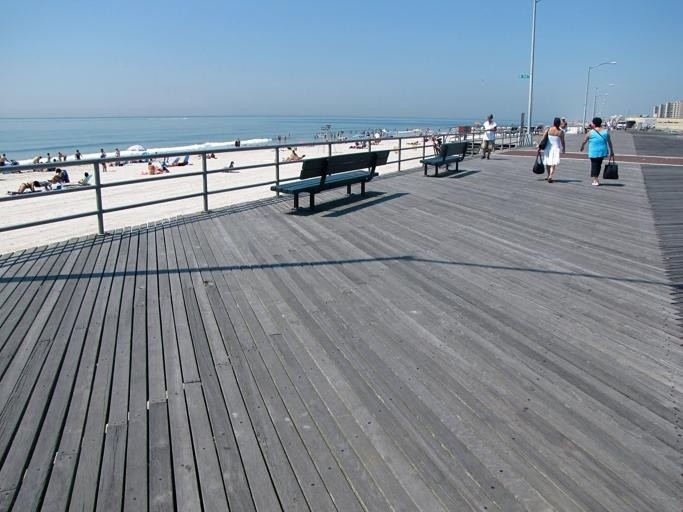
[
  {"x1": 533, "y1": 152, "x2": 544, "y2": 174},
  {"x1": 603, "y1": 157, "x2": 618, "y2": 179},
  {"x1": 539, "y1": 131, "x2": 548, "y2": 150}
]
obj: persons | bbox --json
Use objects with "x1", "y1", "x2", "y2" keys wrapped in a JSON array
[
  {"x1": 314, "y1": 128, "x2": 443, "y2": 156},
  {"x1": 478, "y1": 113, "x2": 498, "y2": 159},
  {"x1": 235, "y1": 138, "x2": 240, "y2": 147},
  {"x1": 229, "y1": 161, "x2": 234, "y2": 167},
  {"x1": 561, "y1": 117, "x2": 568, "y2": 134},
  {"x1": 0, "y1": 148, "x2": 215, "y2": 195},
  {"x1": 535, "y1": 117, "x2": 566, "y2": 183},
  {"x1": 277, "y1": 132, "x2": 306, "y2": 161},
  {"x1": 580, "y1": 117, "x2": 614, "y2": 186}
]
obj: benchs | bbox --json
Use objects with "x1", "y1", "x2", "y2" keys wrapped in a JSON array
[
  {"x1": 271, "y1": 147, "x2": 391, "y2": 211},
  {"x1": 420, "y1": 142, "x2": 469, "y2": 176}
]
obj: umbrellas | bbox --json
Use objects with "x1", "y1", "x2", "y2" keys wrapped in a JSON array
[{"x1": 127, "y1": 144, "x2": 145, "y2": 151}]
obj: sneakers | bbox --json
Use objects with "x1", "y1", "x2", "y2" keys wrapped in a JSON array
[
  {"x1": 592, "y1": 180, "x2": 599, "y2": 186},
  {"x1": 481, "y1": 156, "x2": 490, "y2": 159},
  {"x1": 545, "y1": 178, "x2": 552, "y2": 183}
]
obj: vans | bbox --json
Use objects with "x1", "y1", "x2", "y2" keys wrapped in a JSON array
[{"x1": 617, "y1": 121, "x2": 626, "y2": 130}]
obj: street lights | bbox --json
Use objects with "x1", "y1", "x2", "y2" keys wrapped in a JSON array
[{"x1": 583, "y1": 62, "x2": 616, "y2": 132}]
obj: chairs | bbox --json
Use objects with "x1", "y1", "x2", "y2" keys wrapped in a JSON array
[{"x1": 62, "y1": 175, "x2": 92, "y2": 189}]
